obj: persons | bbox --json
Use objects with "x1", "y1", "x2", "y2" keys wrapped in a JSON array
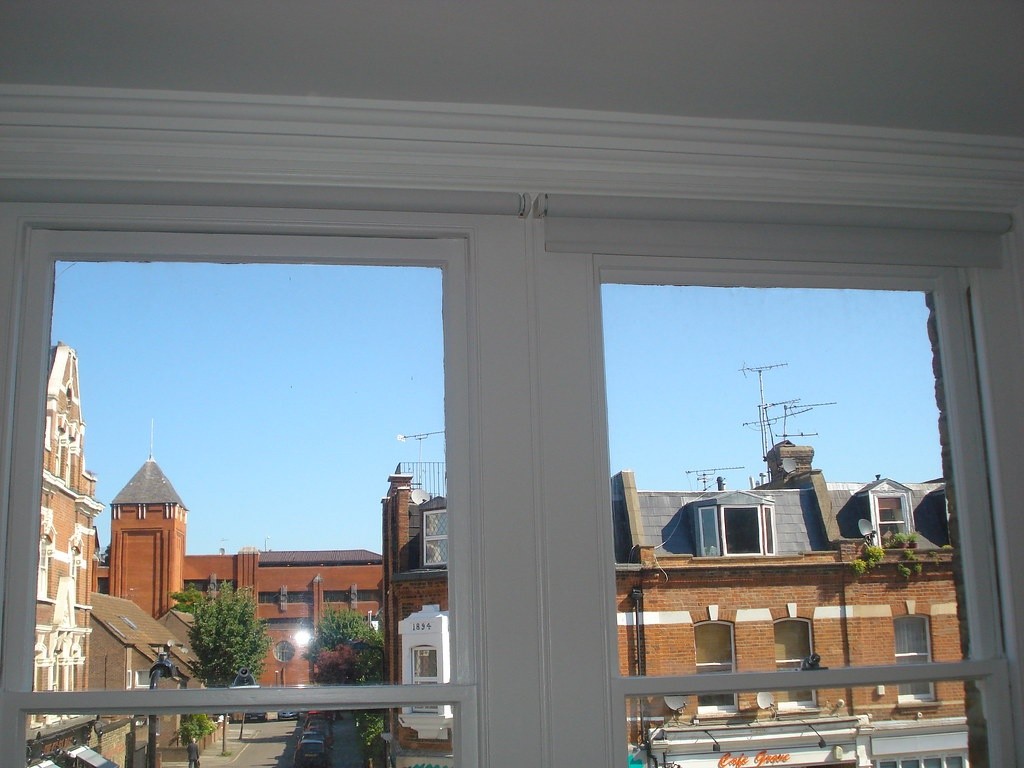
[{"x1": 186, "y1": 738, "x2": 200, "y2": 768}]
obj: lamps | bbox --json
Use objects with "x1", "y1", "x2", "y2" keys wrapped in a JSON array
[
  {"x1": 704, "y1": 730, "x2": 721, "y2": 752},
  {"x1": 808, "y1": 724, "x2": 826, "y2": 748}
]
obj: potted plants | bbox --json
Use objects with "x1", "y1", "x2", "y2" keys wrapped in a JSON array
[{"x1": 890, "y1": 532, "x2": 921, "y2": 548}]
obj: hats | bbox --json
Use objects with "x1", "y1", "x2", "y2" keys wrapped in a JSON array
[{"x1": 191, "y1": 737, "x2": 196, "y2": 742}]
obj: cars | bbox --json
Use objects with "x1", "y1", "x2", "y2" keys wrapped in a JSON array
[
  {"x1": 244, "y1": 711, "x2": 268, "y2": 724},
  {"x1": 294, "y1": 738, "x2": 335, "y2": 768},
  {"x1": 277, "y1": 711, "x2": 299, "y2": 721},
  {"x1": 299, "y1": 711, "x2": 336, "y2": 751}
]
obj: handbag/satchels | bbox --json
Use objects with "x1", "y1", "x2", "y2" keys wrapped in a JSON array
[{"x1": 197, "y1": 760, "x2": 200, "y2": 768}]
